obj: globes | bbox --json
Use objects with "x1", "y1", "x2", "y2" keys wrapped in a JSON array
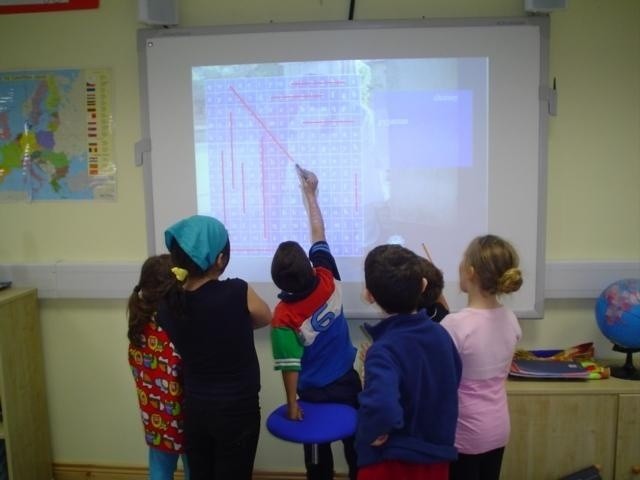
[{"x1": 594, "y1": 278, "x2": 640, "y2": 380}]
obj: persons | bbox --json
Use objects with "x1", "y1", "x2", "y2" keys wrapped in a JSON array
[
  {"x1": 352, "y1": 243, "x2": 464, "y2": 479},
  {"x1": 127, "y1": 253, "x2": 190, "y2": 480},
  {"x1": 270, "y1": 169, "x2": 363, "y2": 480},
  {"x1": 154, "y1": 215, "x2": 271, "y2": 480},
  {"x1": 359, "y1": 255, "x2": 450, "y2": 363},
  {"x1": 439, "y1": 237, "x2": 523, "y2": 480}
]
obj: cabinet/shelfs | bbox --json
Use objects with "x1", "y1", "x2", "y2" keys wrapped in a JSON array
[{"x1": 502, "y1": 359, "x2": 640, "y2": 480}]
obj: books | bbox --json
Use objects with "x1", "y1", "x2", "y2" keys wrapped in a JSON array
[{"x1": 509, "y1": 360, "x2": 589, "y2": 379}]
